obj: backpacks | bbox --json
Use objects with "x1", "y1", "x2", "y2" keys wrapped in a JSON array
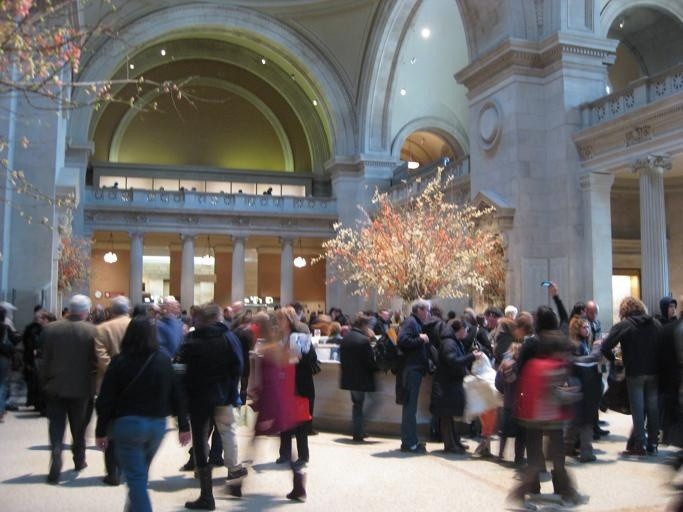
[{"x1": 372, "y1": 326, "x2": 405, "y2": 375}]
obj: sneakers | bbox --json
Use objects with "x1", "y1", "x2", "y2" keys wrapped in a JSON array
[{"x1": 401, "y1": 426, "x2": 661, "y2": 462}]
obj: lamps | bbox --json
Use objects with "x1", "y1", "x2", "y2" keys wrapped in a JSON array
[
  {"x1": 103, "y1": 232, "x2": 118, "y2": 264},
  {"x1": 408, "y1": 142, "x2": 420, "y2": 170},
  {"x1": 293, "y1": 239, "x2": 307, "y2": 269}
]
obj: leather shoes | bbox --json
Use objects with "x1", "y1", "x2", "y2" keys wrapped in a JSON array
[{"x1": 45, "y1": 453, "x2": 309, "y2": 510}]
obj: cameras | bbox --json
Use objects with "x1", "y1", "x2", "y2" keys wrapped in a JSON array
[{"x1": 541, "y1": 281, "x2": 552, "y2": 287}]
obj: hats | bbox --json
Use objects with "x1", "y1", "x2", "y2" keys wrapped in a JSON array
[{"x1": 66, "y1": 291, "x2": 132, "y2": 317}]
